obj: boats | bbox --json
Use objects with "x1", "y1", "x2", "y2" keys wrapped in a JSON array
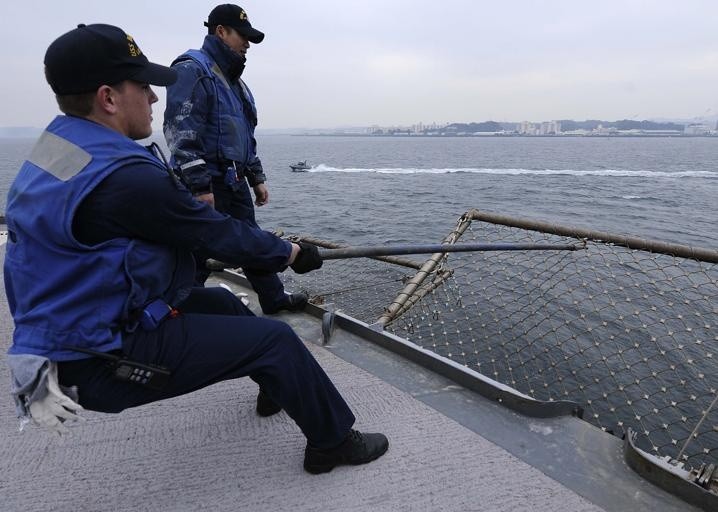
[{"x1": 289, "y1": 160, "x2": 311, "y2": 172}]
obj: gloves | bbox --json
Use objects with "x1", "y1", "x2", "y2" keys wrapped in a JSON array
[
  {"x1": 6, "y1": 354, "x2": 86, "y2": 441},
  {"x1": 292, "y1": 241, "x2": 323, "y2": 274}
]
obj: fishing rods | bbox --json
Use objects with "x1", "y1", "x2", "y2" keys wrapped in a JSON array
[{"x1": 210, "y1": 242, "x2": 589, "y2": 272}]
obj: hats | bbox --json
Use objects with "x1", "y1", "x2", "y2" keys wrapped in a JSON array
[
  {"x1": 203, "y1": 4, "x2": 265, "y2": 44},
  {"x1": 43, "y1": 22, "x2": 178, "y2": 93}
]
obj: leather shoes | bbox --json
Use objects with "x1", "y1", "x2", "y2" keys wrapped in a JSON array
[
  {"x1": 256, "y1": 391, "x2": 281, "y2": 418},
  {"x1": 301, "y1": 432, "x2": 388, "y2": 474},
  {"x1": 263, "y1": 292, "x2": 308, "y2": 315}
]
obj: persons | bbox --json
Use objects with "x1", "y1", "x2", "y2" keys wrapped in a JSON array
[
  {"x1": 1, "y1": 18, "x2": 391, "y2": 476},
  {"x1": 160, "y1": 2, "x2": 311, "y2": 319}
]
